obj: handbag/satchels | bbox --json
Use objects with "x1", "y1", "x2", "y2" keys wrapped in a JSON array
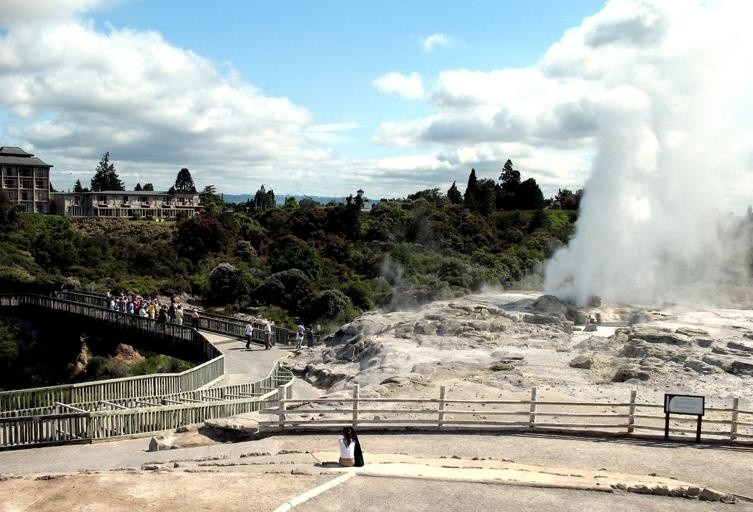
[{"x1": 299, "y1": 333, "x2": 303, "y2": 337}]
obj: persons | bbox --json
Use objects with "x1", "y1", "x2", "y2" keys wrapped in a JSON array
[
  {"x1": 105, "y1": 289, "x2": 200, "y2": 331},
  {"x1": 245, "y1": 320, "x2": 254, "y2": 349},
  {"x1": 262, "y1": 321, "x2": 273, "y2": 350},
  {"x1": 339, "y1": 428, "x2": 355, "y2": 467},
  {"x1": 315, "y1": 321, "x2": 321, "y2": 342},
  {"x1": 306, "y1": 324, "x2": 313, "y2": 347},
  {"x1": 296, "y1": 322, "x2": 305, "y2": 349}
]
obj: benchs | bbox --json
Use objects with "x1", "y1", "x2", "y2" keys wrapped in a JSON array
[{"x1": 286, "y1": 331, "x2": 320, "y2": 346}]
